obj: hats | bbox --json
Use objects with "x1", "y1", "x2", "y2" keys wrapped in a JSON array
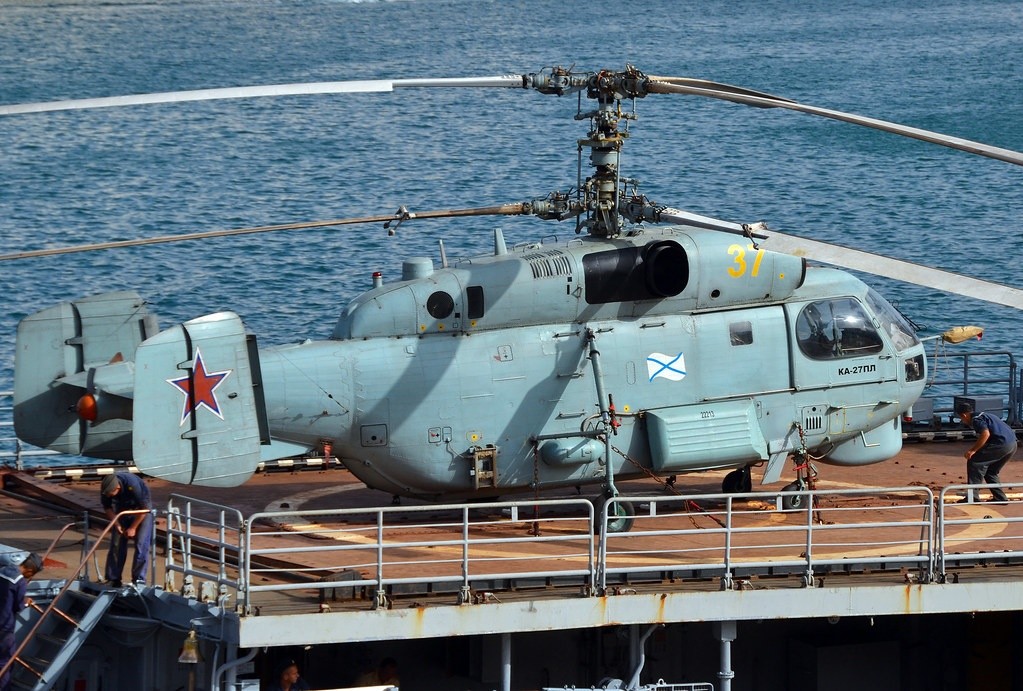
[
  {"x1": 951, "y1": 404, "x2": 972, "y2": 413},
  {"x1": 100, "y1": 474, "x2": 117, "y2": 495},
  {"x1": 30, "y1": 553, "x2": 43, "y2": 571}
]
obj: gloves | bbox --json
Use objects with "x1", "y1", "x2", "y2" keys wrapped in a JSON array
[{"x1": 24, "y1": 597, "x2": 35, "y2": 607}]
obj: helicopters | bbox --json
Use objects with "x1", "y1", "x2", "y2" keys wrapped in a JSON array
[{"x1": 1, "y1": 65, "x2": 1023, "y2": 534}]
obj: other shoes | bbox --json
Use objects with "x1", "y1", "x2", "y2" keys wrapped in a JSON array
[
  {"x1": 99, "y1": 579, "x2": 122, "y2": 587},
  {"x1": 956, "y1": 495, "x2": 979, "y2": 505},
  {"x1": 986, "y1": 499, "x2": 1009, "y2": 505}
]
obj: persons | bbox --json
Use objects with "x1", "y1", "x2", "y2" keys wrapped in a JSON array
[
  {"x1": 99, "y1": 472, "x2": 153, "y2": 587},
  {"x1": 957, "y1": 402, "x2": 1018, "y2": 505},
  {"x1": 0, "y1": 552, "x2": 43, "y2": 688},
  {"x1": 349, "y1": 657, "x2": 400, "y2": 687},
  {"x1": 265, "y1": 658, "x2": 310, "y2": 691}
]
